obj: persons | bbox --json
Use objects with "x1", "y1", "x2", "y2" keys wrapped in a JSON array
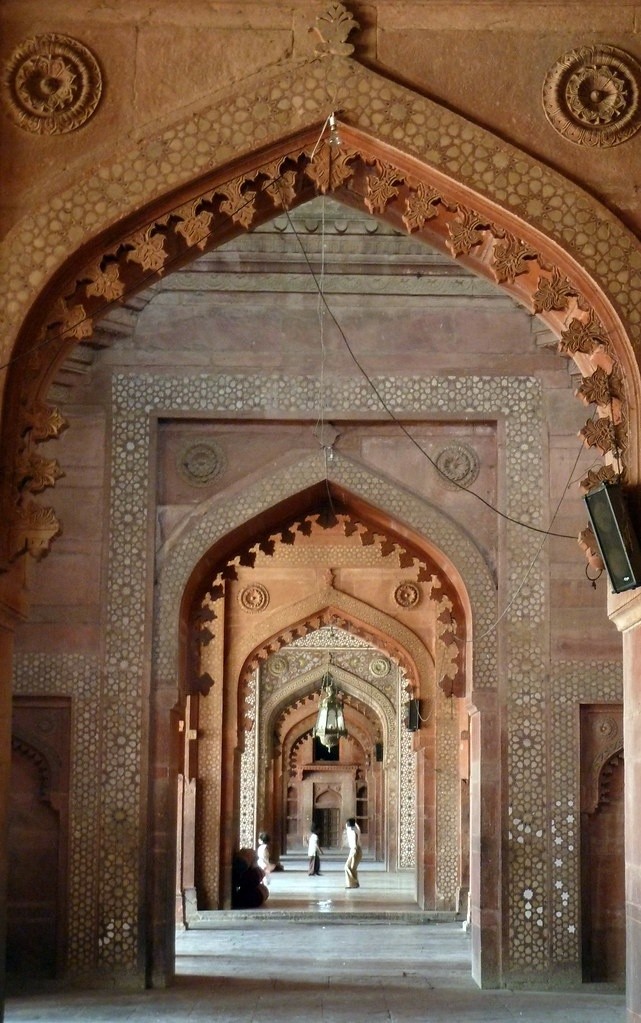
[
  {"x1": 257, "y1": 833, "x2": 277, "y2": 885},
  {"x1": 308, "y1": 824, "x2": 324, "y2": 875},
  {"x1": 344, "y1": 818, "x2": 362, "y2": 889}
]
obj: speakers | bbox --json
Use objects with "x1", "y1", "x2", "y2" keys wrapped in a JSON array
[
  {"x1": 375, "y1": 744, "x2": 383, "y2": 762},
  {"x1": 581, "y1": 478, "x2": 641, "y2": 596},
  {"x1": 405, "y1": 698, "x2": 421, "y2": 733}
]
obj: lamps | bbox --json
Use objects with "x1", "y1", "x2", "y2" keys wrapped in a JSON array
[{"x1": 312, "y1": 625, "x2": 348, "y2": 752}]
